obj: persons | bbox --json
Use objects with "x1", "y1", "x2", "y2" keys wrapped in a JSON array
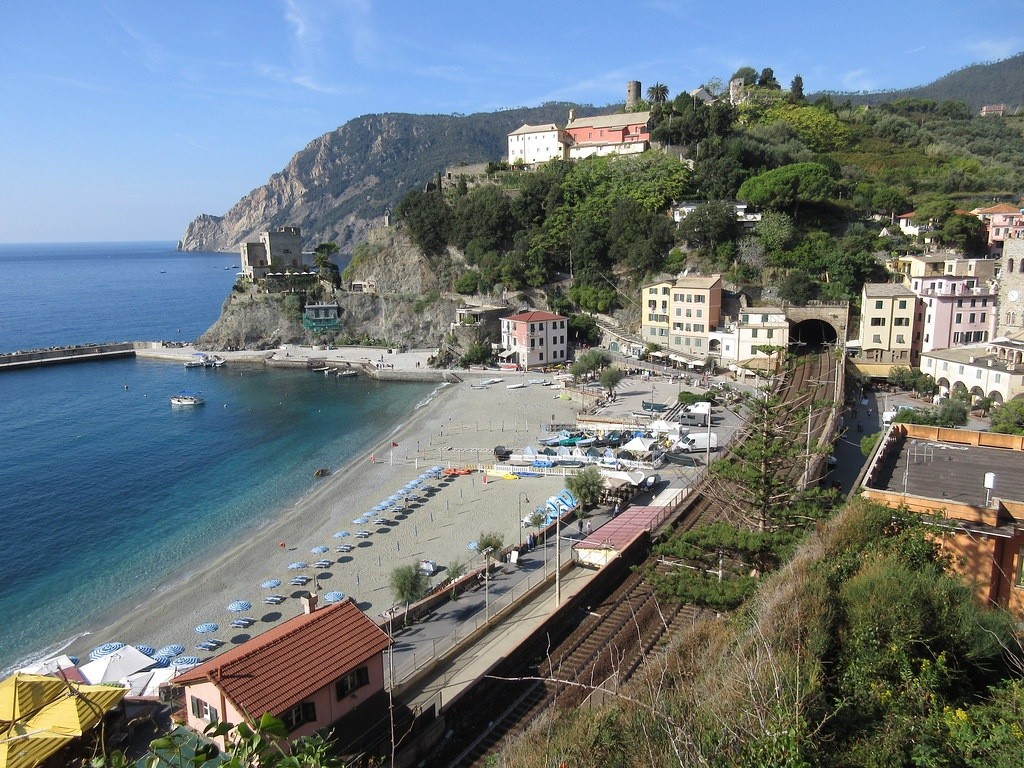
[
  {"x1": 525, "y1": 532, "x2": 536, "y2": 551},
  {"x1": 587, "y1": 519, "x2": 592, "y2": 535},
  {"x1": 577, "y1": 518, "x2": 583, "y2": 535}
]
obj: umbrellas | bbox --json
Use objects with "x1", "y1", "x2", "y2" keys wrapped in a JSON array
[
  {"x1": 288, "y1": 562, "x2": 307, "y2": 579},
  {"x1": 363, "y1": 512, "x2": 378, "y2": 523},
  {"x1": 417, "y1": 466, "x2": 444, "y2": 488},
  {"x1": 380, "y1": 500, "x2": 396, "y2": 518},
  {"x1": 353, "y1": 517, "x2": 369, "y2": 531},
  {"x1": 333, "y1": 531, "x2": 350, "y2": 545},
  {"x1": 467, "y1": 541, "x2": 479, "y2": 556},
  {"x1": 227, "y1": 600, "x2": 252, "y2": 620},
  {"x1": 324, "y1": 591, "x2": 346, "y2": 607},
  {"x1": 14, "y1": 642, "x2": 205, "y2": 698},
  {"x1": 372, "y1": 505, "x2": 387, "y2": 518},
  {"x1": 311, "y1": 546, "x2": 329, "y2": 561},
  {"x1": 196, "y1": 623, "x2": 219, "y2": 642},
  {"x1": 260, "y1": 580, "x2": 281, "y2": 596},
  {"x1": 388, "y1": 480, "x2": 423, "y2": 508}
]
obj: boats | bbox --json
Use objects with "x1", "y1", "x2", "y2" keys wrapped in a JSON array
[
  {"x1": 314, "y1": 366, "x2": 329, "y2": 372},
  {"x1": 183, "y1": 351, "x2": 226, "y2": 369},
  {"x1": 170, "y1": 392, "x2": 209, "y2": 405},
  {"x1": 324, "y1": 367, "x2": 339, "y2": 374},
  {"x1": 338, "y1": 370, "x2": 358, "y2": 378}
]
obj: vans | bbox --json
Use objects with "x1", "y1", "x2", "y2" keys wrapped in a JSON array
[{"x1": 678, "y1": 432, "x2": 722, "y2": 453}]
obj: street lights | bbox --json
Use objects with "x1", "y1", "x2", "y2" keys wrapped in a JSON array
[{"x1": 518, "y1": 491, "x2": 530, "y2": 552}]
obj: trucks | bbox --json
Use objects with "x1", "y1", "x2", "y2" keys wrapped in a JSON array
[{"x1": 677, "y1": 400, "x2": 713, "y2": 427}]
